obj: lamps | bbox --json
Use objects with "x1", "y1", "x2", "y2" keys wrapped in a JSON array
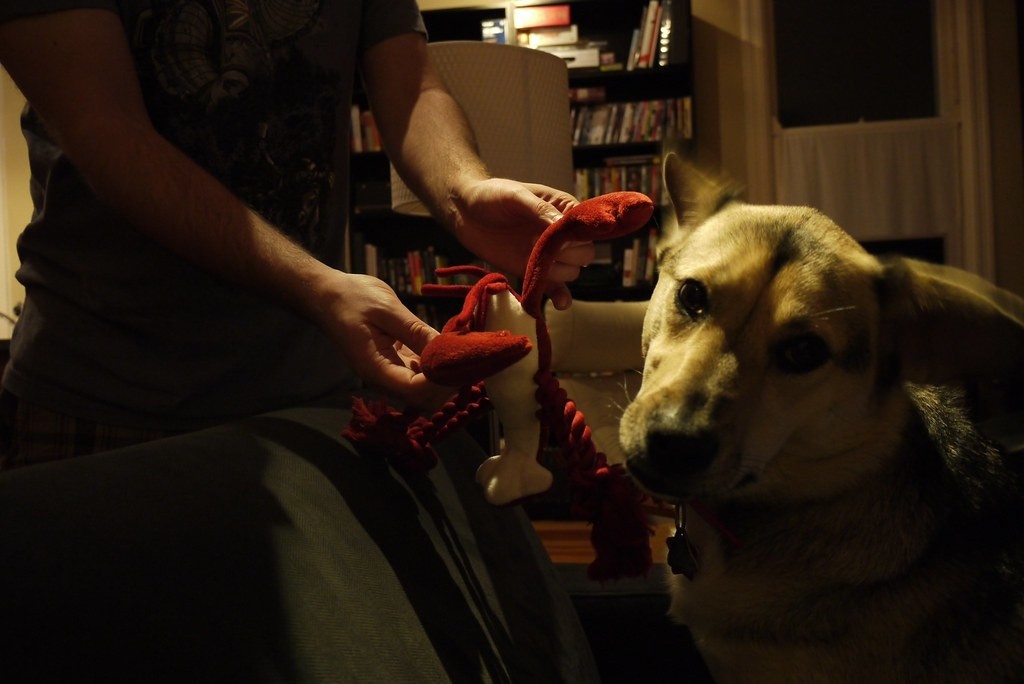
[{"x1": 390, "y1": 40, "x2": 574, "y2": 456}]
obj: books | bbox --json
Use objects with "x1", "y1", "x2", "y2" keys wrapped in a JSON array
[{"x1": 348, "y1": 1, "x2": 693, "y2": 298}]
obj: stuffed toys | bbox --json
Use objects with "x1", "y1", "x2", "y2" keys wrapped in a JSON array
[{"x1": 342, "y1": 190, "x2": 655, "y2": 579}]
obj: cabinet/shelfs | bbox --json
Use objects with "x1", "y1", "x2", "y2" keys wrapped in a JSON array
[{"x1": 338, "y1": 0, "x2": 694, "y2": 336}]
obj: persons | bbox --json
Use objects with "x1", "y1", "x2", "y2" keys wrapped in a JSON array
[{"x1": 0, "y1": 0, "x2": 594, "y2": 473}]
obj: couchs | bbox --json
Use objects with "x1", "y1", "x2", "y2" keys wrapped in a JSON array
[{"x1": 0, "y1": 302, "x2": 713, "y2": 684}]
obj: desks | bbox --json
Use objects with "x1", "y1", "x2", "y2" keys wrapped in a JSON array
[{"x1": 529, "y1": 515, "x2": 677, "y2": 592}]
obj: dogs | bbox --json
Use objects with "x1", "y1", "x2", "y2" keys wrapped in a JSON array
[{"x1": 618, "y1": 149, "x2": 1024, "y2": 684}]
